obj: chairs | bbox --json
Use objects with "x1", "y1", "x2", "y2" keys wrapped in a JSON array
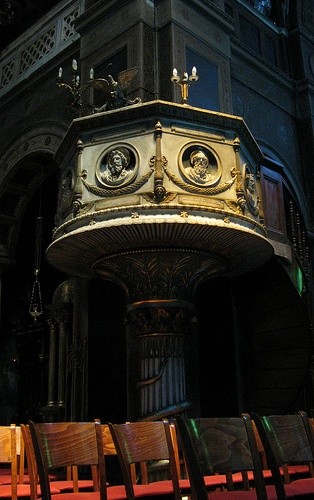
[{"x1": 0, "y1": 412, "x2": 313, "y2": 500}]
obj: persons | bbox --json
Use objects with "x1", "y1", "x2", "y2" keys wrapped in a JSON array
[
  {"x1": 185, "y1": 150, "x2": 213, "y2": 184},
  {"x1": 246, "y1": 174, "x2": 257, "y2": 206},
  {"x1": 100, "y1": 150, "x2": 132, "y2": 186}
]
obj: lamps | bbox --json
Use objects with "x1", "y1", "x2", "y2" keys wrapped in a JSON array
[
  {"x1": 171, "y1": 66, "x2": 198, "y2": 103},
  {"x1": 29, "y1": 216, "x2": 43, "y2": 321},
  {"x1": 56, "y1": 60, "x2": 95, "y2": 104}
]
